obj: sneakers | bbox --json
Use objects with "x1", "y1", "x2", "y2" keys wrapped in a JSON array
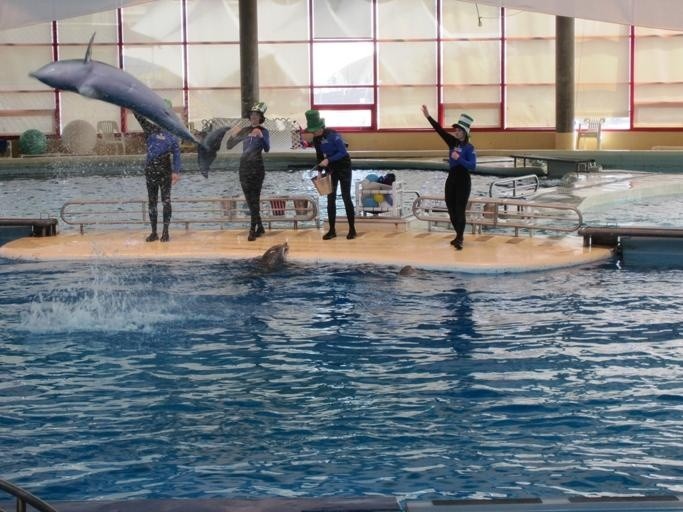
[
  {"x1": 347, "y1": 230, "x2": 357, "y2": 239},
  {"x1": 146, "y1": 233, "x2": 158, "y2": 241},
  {"x1": 323, "y1": 232, "x2": 337, "y2": 239},
  {"x1": 451, "y1": 239, "x2": 464, "y2": 248},
  {"x1": 160, "y1": 234, "x2": 169, "y2": 242},
  {"x1": 248, "y1": 227, "x2": 264, "y2": 241}
]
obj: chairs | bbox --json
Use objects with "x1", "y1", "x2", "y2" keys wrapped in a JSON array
[
  {"x1": 94, "y1": 121, "x2": 125, "y2": 155},
  {"x1": 577, "y1": 117, "x2": 606, "y2": 150}
]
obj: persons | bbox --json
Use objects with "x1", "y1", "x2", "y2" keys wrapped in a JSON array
[
  {"x1": 227, "y1": 102, "x2": 270, "y2": 241},
  {"x1": 304, "y1": 110, "x2": 356, "y2": 240},
  {"x1": 361, "y1": 173, "x2": 396, "y2": 217},
  {"x1": 420, "y1": 105, "x2": 476, "y2": 250},
  {"x1": 132, "y1": 111, "x2": 180, "y2": 242}
]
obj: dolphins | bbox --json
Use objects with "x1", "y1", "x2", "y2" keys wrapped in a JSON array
[
  {"x1": 29, "y1": 31, "x2": 232, "y2": 180},
  {"x1": 244, "y1": 241, "x2": 291, "y2": 278},
  {"x1": 398, "y1": 264, "x2": 415, "y2": 276}
]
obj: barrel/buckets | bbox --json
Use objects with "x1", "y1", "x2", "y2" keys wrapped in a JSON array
[
  {"x1": 270, "y1": 200, "x2": 284, "y2": 216},
  {"x1": 294, "y1": 199, "x2": 308, "y2": 216},
  {"x1": 222, "y1": 201, "x2": 236, "y2": 216},
  {"x1": 309, "y1": 164, "x2": 333, "y2": 196}
]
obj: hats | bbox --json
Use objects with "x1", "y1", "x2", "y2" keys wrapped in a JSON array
[
  {"x1": 249, "y1": 103, "x2": 267, "y2": 123},
  {"x1": 305, "y1": 110, "x2": 325, "y2": 132},
  {"x1": 453, "y1": 113, "x2": 474, "y2": 132}
]
